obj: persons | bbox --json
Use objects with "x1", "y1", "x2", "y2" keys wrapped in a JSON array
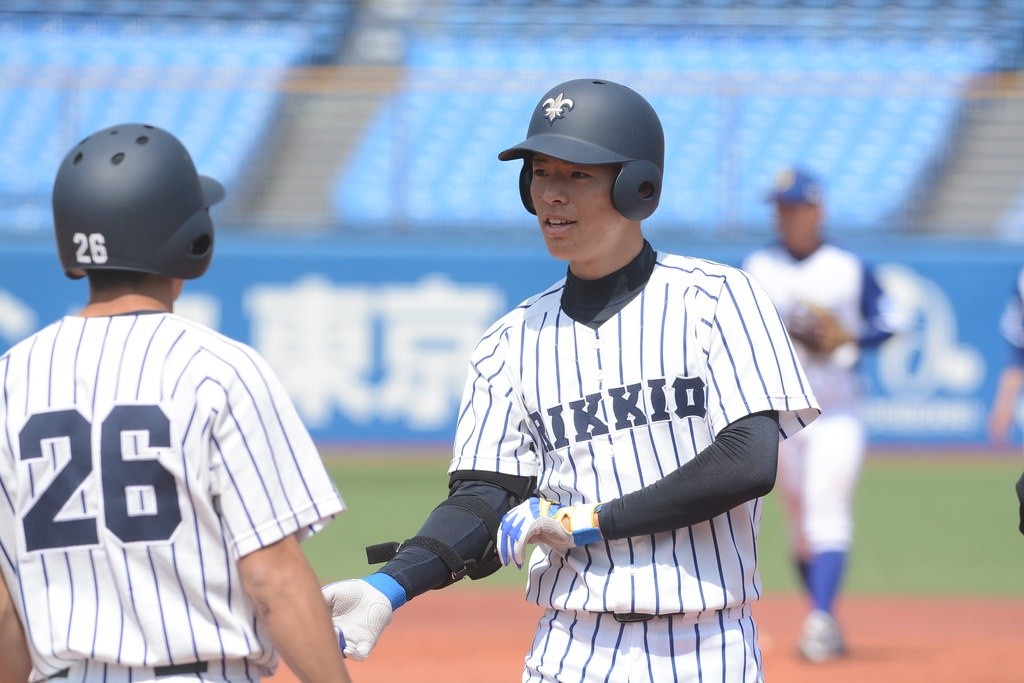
[
  {"x1": 991, "y1": 263, "x2": 1024, "y2": 535},
  {"x1": 320, "y1": 78, "x2": 823, "y2": 683},
  {"x1": 742, "y1": 166, "x2": 894, "y2": 665},
  {"x1": 0, "y1": 124, "x2": 350, "y2": 683}
]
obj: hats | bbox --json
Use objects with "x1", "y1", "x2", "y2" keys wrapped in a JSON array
[{"x1": 762, "y1": 164, "x2": 823, "y2": 204}]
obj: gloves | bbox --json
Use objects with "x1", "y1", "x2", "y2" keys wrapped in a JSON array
[
  {"x1": 496, "y1": 497, "x2": 603, "y2": 570},
  {"x1": 320, "y1": 573, "x2": 407, "y2": 661}
]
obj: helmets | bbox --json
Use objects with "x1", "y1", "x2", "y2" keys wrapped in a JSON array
[
  {"x1": 51, "y1": 123, "x2": 225, "y2": 280},
  {"x1": 498, "y1": 78, "x2": 664, "y2": 221}
]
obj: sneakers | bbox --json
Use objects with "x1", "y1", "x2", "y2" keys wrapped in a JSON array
[{"x1": 799, "y1": 609, "x2": 844, "y2": 665}]
